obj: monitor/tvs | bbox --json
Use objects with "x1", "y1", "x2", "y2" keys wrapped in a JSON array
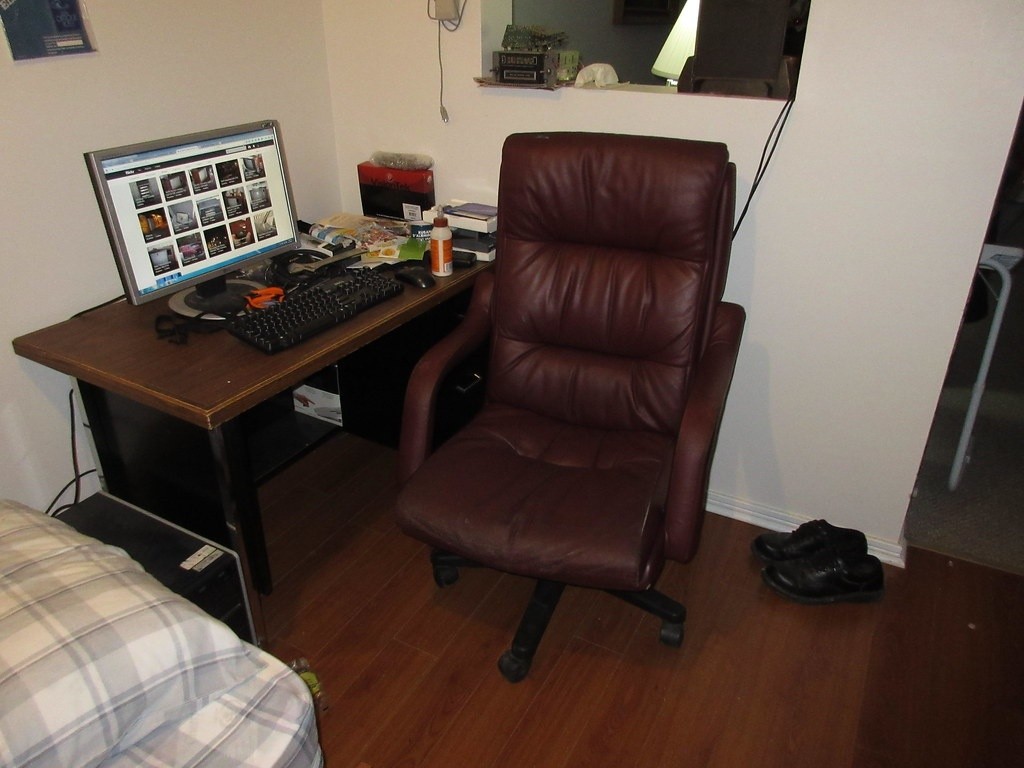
[{"x1": 83, "y1": 120, "x2": 302, "y2": 320}]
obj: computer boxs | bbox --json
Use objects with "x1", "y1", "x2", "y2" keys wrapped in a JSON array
[{"x1": 55, "y1": 492, "x2": 259, "y2": 648}]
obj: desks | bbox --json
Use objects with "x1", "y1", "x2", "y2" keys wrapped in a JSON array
[{"x1": 12, "y1": 234, "x2": 497, "y2": 598}]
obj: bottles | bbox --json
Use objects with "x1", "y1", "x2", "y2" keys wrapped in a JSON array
[{"x1": 430, "y1": 203, "x2": 454, "y2": 277}]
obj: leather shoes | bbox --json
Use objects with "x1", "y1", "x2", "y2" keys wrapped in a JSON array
[
  {"x1": 762, "y1": 551, "x2": 886, "y2": 605},
  {"x1": 755, "y1": 519, "x2": 866, "y2": 564}
]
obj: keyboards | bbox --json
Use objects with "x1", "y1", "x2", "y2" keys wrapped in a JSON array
[{"x1": 228, "y1": 266, "x2": 405, "y2": 355}]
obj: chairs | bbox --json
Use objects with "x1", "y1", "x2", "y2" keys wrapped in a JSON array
[{"x1": 391, "y1": 132, "x2": 747, "y2": 683}]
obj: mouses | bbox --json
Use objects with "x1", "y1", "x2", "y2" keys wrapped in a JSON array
[{"x1": 394, "y1": 266, "x2": 435, "y2": 289}]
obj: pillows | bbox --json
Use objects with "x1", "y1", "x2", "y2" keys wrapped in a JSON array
[{"x1": 1, "y1": 497, "x2": 258, "y2": 768}]
